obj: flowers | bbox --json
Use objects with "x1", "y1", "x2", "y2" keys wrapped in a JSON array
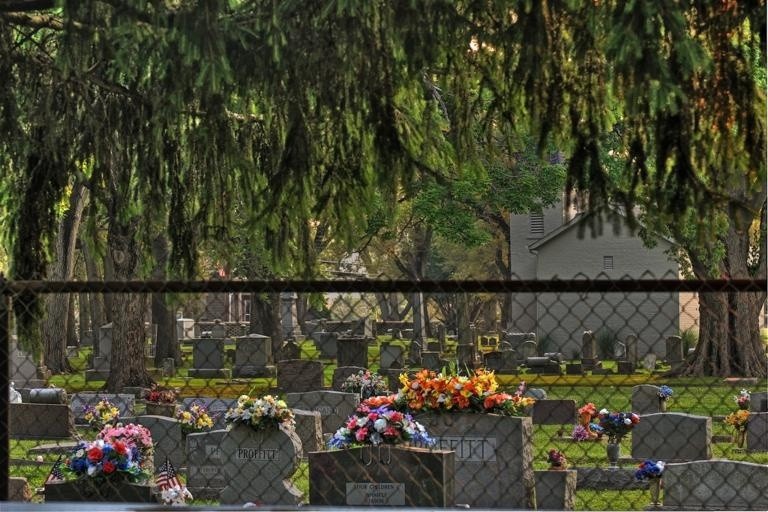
[
  {"x1": 324, "y1": 368, "x2": 538, "y2": 449},
  {"x1": 547, "y1": 384, "x2": 752, "y2": 480},
  {"x1": 52, "y1": 380, "x2": 298, "y2": 483}
]
